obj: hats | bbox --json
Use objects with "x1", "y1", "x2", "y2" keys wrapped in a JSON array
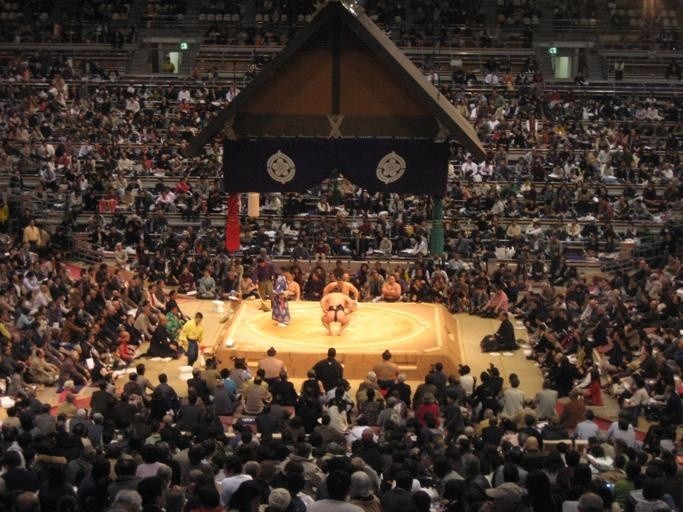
[
  {"x1": 268, "y1": 486, "x2": 292, "y2": 510},
  {"x1": 421, "y1": 391, "x2": 436, "y2": 405},
  {"x1": 484, "y1": 481, "x2": 525, "y2": 502},
  {"x1": 523, "y1": 436, "x2": 541, "y2": 450}
]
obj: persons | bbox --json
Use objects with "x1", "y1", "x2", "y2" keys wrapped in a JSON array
[
  {"x1": 320, "y1": 286, "x2": 355, "y2": 336},
  {"x1": 1, "y1": 2, "x2": 204, "y2": 368},
  {"x1": 0, "y1": 348, "x2": 681, "y2": 510},
  {"x1": 252, "y1": 255, "x2": 275, "y2": 310},
  {"x1": 272, "y1": 271, "x2": 294, "y2": 327},
  {"x1": 199, "y1": 1, "x2": 362, "y2": 300},
  {"x1": 324, "y1": 272, "x2": 358, "y2": 313},
  {"x1": 352, "y1": 0, "x2": 681, "y2": 357}
]
